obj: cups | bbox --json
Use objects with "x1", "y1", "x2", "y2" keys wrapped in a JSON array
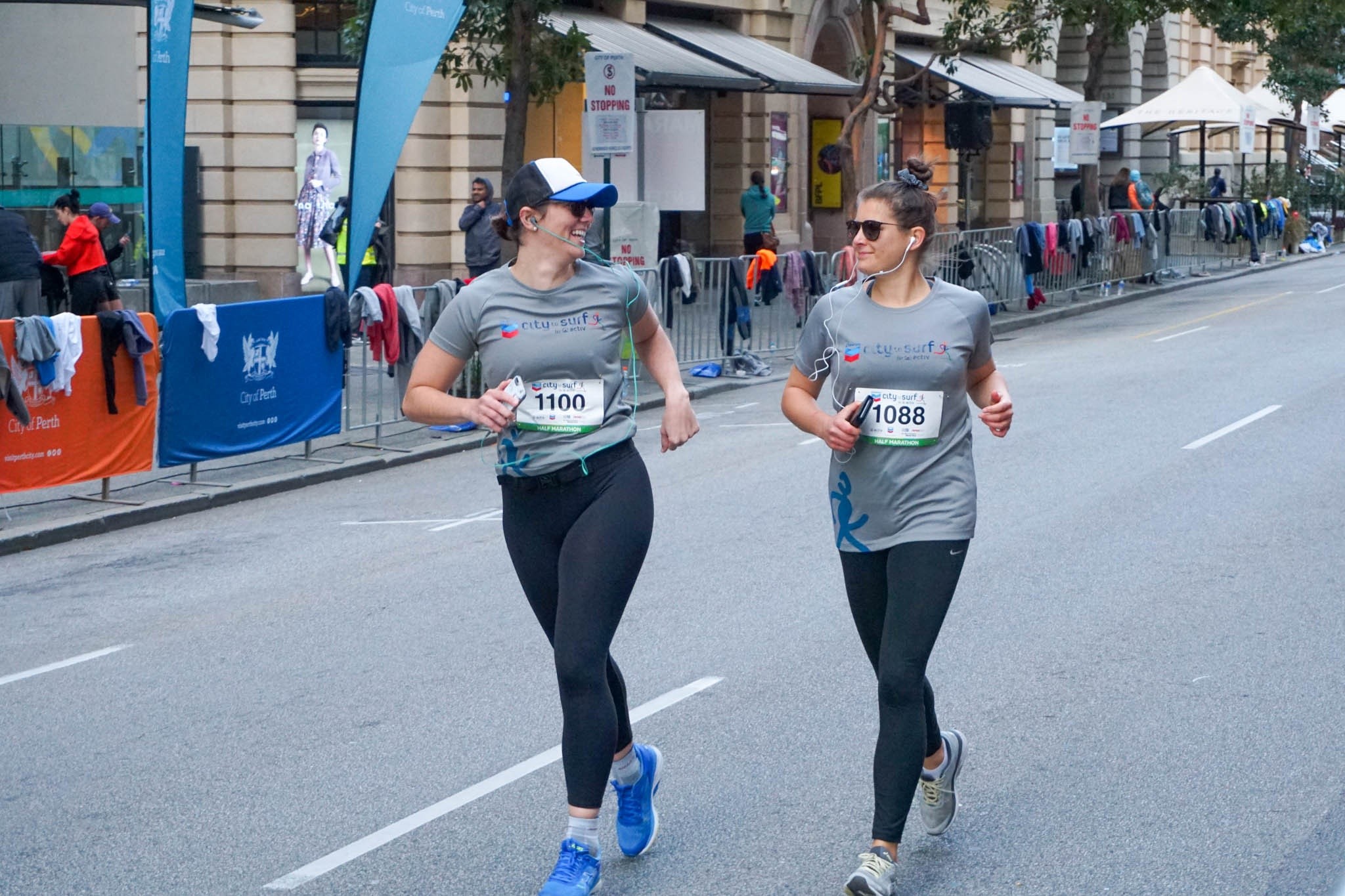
[{"x1": 736, "y1": 370, "x2": 746, "y2": 376}]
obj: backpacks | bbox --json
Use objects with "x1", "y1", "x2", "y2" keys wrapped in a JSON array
[
  {"x1": 1136, "y1": 181, "x2": 1153, "y2": 207},
  {"x1": 1210, "y1": 178, "x2": 1220, "y2": 197}
]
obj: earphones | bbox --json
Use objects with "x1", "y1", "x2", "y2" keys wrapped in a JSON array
[
  {"x1": 531, "y1": 218, "x2": 538, "y2": 227},
  {"x1": 910, "y1": 236, "x2": 916, "y2": 245}
]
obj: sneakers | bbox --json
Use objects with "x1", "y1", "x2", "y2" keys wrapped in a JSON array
[
  {"x1": 844, "y1": 847, "x2": 898, "y2": 896},
  {"x1": 610, "y1": 743, "x2": 665, "y2": 859},
  {"x1": 537, "y1": 839, "x2": 601, "y2": 896},
  {"x1": 919, "y1": 728, "x2": 968, "y2": 836}
]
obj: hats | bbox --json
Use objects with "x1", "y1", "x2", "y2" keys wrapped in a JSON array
[
  {"x1": 502, "y1": 158, "x2": 619, "y2": 224},
  {"x1": 1130, "y1": 169, "x2": 1141, "y2": 182},
  {"x1": 89, "y1": 202, "x2": 121, "y2": 225}
]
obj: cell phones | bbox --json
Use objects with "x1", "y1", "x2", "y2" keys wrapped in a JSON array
[
  {"x1": 849, "y1": 397, "x2": 873, "y2": 429},
  {"x1": 498, "y1": 376, "x2": 527, "y2": 416}
]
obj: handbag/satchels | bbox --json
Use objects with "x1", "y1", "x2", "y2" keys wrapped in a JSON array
[{"x1": 762, "y1": 232, "x2": 781, "y2": 250}]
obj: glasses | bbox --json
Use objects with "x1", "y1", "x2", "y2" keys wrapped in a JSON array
[
  {"x1": 567, "y1": 201, "x2": 596, "y2": 218},
  {"x1": 844, "y1": 219, "x2": 904, "y2": 241}
]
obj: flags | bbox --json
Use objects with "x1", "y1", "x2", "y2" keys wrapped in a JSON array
[
  {"x1": 148, "y1": 1, "x2": 194, "y2": 327},
  {"x1": 348, "y1": 0, "x2": 460, "y2": 292}
]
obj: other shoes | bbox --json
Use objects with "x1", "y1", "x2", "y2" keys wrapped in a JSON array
[
  {"x1": 300, "y1": 272, "x2": 314, "y2": 284},
  {"x1": 330, "y1": 274, "x2": 341, "y2": 288}
]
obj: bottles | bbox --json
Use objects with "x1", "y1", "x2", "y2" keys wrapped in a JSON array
[
  {"x1": 1260, "y1": 251, "x2": 1266, "y2": 264},
  {"x1": 771, "y1": 342, "x2": 777, "y2": 363},
  {"x1": 620, "y1": 367, "x2": 630, "y2": 400},
  {"x1": 1104, "y1": 280, "x2": 1111, "y2": 297},
  {"x1": 1117, "y1": 277, "x2": 1125, "y2": 295},
  {"x1": 1282, "y1": 247, "x2": 1287, "y2": 261},
  {"x1": 725, "y1": 359, "x2": 732, "y2": 376}
]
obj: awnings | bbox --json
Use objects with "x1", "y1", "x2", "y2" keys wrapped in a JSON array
[
  {"x1": 538, "y1": 0, "x2": 863, "y2": 104},
  {"x1": 890, "y1": 42, "x2": 1085, "y2": 112}
]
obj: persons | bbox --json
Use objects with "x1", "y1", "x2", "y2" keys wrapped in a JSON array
[
  {"x1": 1107, "y1": 167, "x2": 1143, "y2": 246},
  {"x1": 458, "y1": 175, "x2": 504, "y2": 278},
  {"x1": 741, "y1": 169, "x2": 775, "y2": 306},
  {"x1": 0, "y1": 206, "x2": 43, "y2": 320},
  {"x1": 1154, "y1": 173, "x2": 1189, "y2": 212},
  {"x1": 1206, "y1": 168, "x2": 1226, "y2": 206},
  {"x1": 781, "y1": 157, "x2": 1013, "y2": 895},
  {"x1": 295, "y1": 122, "x2": 341, "y2": 287},
  {"x1": 1129, "y1": 170, "x2": 1155, "y2": 249},
  {"x1": 335, "y1": 194, "x2": 385, "y2": 345},
  {"x1": 38, "y1": 189, "x2": 108, "y2": 316},
  {"x1": 1069, "y1": 176, "x2": 1103, "y2": 268},
  {"x1": 86, "y1": 202, "x2": 131, "y2": 313},
  {"x1": 402, "y1": 158, "x2": 700, "y2": 896}
]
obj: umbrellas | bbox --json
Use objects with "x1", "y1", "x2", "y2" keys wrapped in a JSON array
[{"x1": 1101, "y1": 65, "x2": 1320, "y2": 206}]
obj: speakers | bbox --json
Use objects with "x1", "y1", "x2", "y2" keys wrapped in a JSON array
[{"x1": 944, "y1": 100, "x2": 993, "y2": 150}]
